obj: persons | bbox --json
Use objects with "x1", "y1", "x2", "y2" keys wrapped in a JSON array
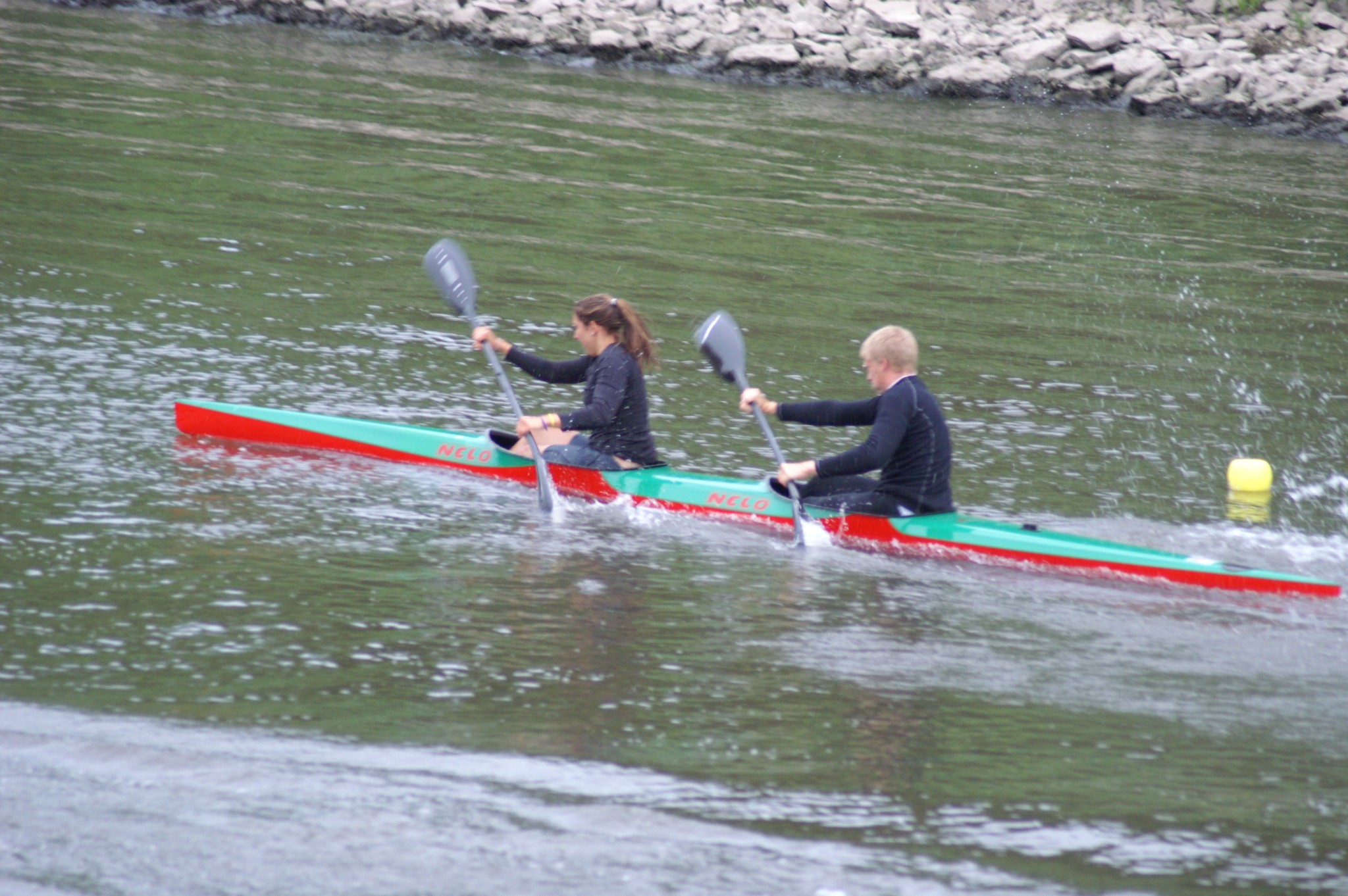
[
  {"x1": 739, "y1": 326, "x2": 953, "y2": 519},
  {"x1": 471, "y1": 293, "x2": 658, "y2": 472}
]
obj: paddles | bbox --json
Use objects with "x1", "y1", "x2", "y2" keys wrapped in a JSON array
[
  {"x1": 421, "y1": 235, "x2": 560, "y2": 513},
  {"x1": 690, "y1": 306, "x2": 812, "y2": 547}
]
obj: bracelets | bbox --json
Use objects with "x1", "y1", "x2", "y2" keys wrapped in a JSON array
[
  {"x1": 540, "y1": 414, "x2": 548, "y2": 430},
  {"x1": 547, "y1": 412, "x2": 554, "y2": 428}
]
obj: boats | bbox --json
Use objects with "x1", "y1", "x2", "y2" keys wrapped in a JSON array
[{"x1": 173, "y1": 397, "x2": 1343, "y2": 599}]
